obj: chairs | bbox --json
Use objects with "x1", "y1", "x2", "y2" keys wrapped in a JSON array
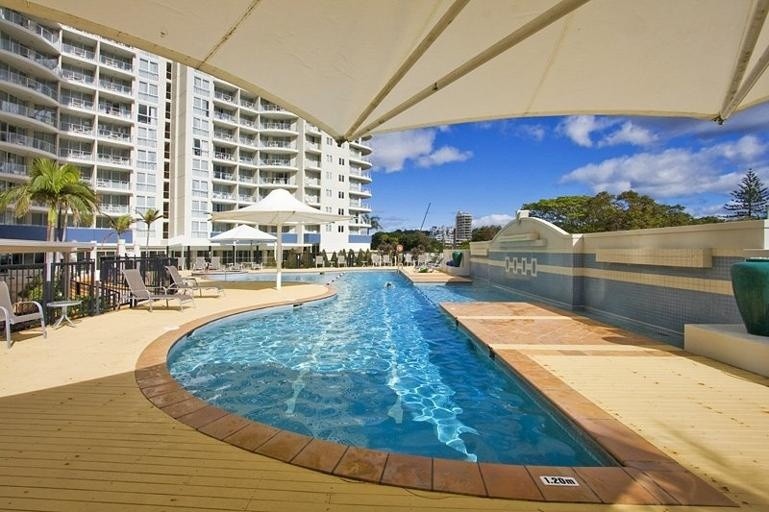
[
  {"x1": 0, "y1": 281, "x2": 47, "y2": 348},
  {"x1": 314, "y1": 254, "x2": 443, "y2": 273},
  {"x1": 121, "y1": 269, "x2": 196, "y2": 312},
  {"x1": 165, "y1": 266, "x2": 223, "y2": 296},
  {"x1": 175, "y1": 254, "x2": 264, "y2": 273}
]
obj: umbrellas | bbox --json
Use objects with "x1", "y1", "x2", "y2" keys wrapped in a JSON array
[
  {"x1": 0, "y1": 1, "x2": 769, "y2": 149},
  {"x1": 209, "y1": 224, "x2": 278, "y2": 245},
  {"x1": 207, "y1": 187, "x2": 361, "y2": 292}
]
